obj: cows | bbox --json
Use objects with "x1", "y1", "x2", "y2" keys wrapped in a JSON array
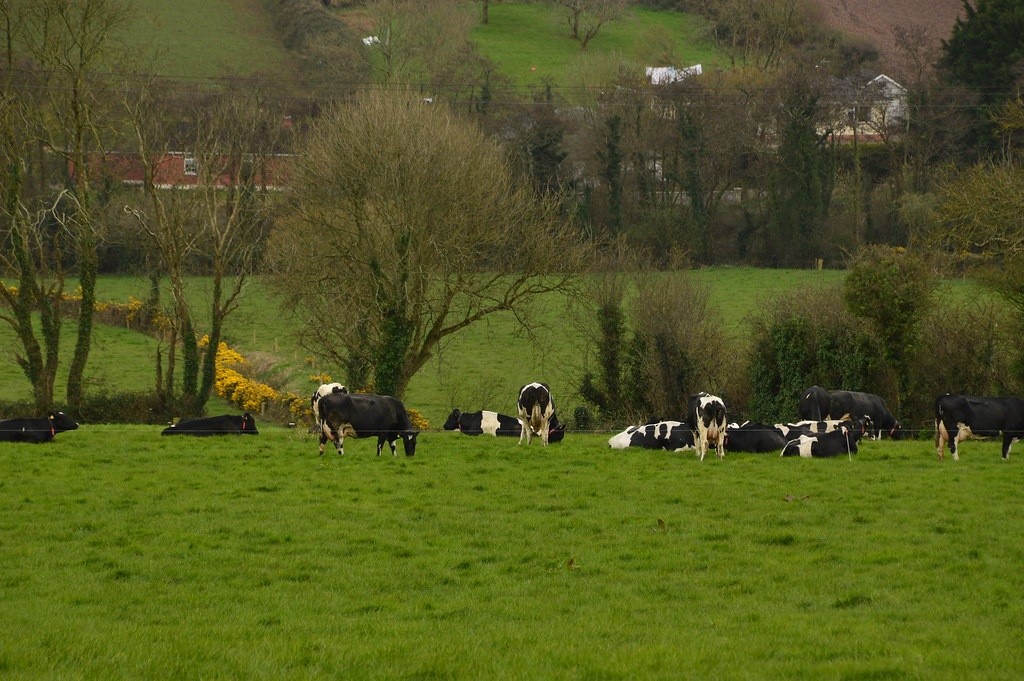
[
  {"x1": 160, "y1": 411, "x2": 260, "y2": 437},
  {"x1": 606, "y1": 386, "x2": 902, "y2": 462},
  {"x1": 0, "y1": 410, "x2": 79, "y2": 445},
  {"x1": 443, "y1": 381, "x2": 565, "y2": 447},
  {"x1": 311, "y1": 382, "x2": 420, "y2": 456},
  {"x1": 934, "y1": 393, "x2": 1024, "y2": 463}
]
